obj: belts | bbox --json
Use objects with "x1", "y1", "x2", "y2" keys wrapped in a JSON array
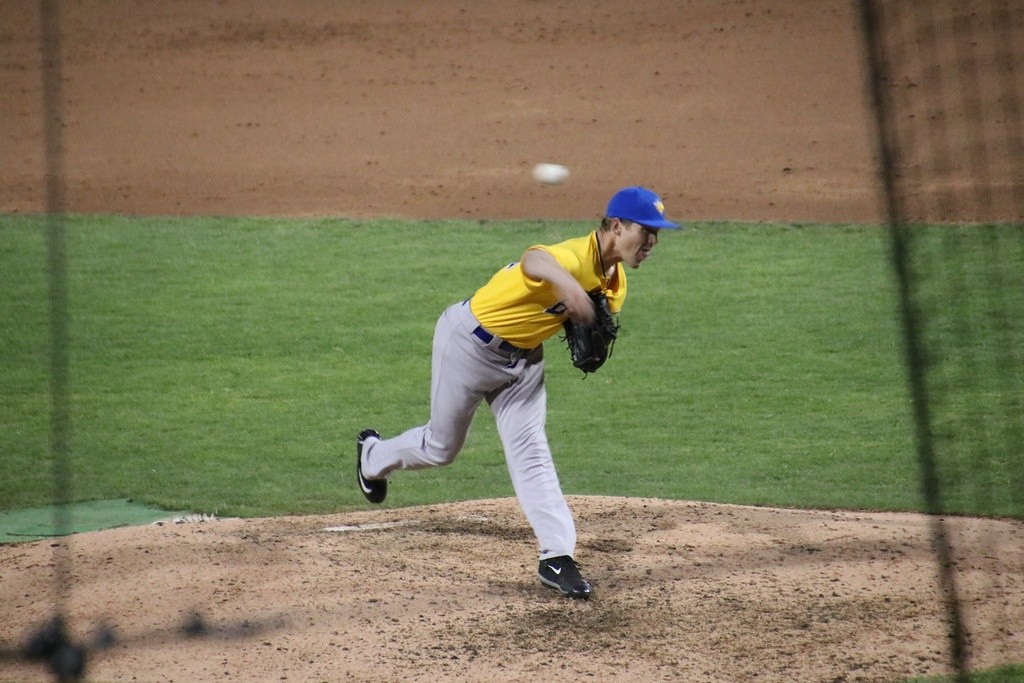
[{"x1": 462, "y1": 297, "x2": 531, "y2": 353}]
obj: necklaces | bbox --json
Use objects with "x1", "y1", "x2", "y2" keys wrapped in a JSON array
[{"x1": 595, "y1": 231, "x2": 611, "y2": 289}]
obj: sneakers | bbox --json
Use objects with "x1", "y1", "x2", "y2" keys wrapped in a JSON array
[
  {"x1": 537, "y1": 558, "x2": 592, "y2": 597},
  {"x1": 356, "y1": 429, "x2": 387, "y2": 504}
]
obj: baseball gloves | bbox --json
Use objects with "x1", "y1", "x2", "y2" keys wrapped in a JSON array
[{"x1": 560, "y1": 295, "x2": 618, "y2": 373}]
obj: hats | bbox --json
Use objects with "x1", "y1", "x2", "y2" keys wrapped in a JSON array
[{"x1": 606, "y1": 186, "x2": 683, "y2": 228}]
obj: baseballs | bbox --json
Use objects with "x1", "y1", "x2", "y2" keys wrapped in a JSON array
[{"x1": 532, "y1": 162, "x2": 570, "y2": 185}]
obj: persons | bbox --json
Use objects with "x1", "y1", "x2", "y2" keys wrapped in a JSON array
[{"x1": 355, "y1": 186, "x2": 682, "y2": 600}]
